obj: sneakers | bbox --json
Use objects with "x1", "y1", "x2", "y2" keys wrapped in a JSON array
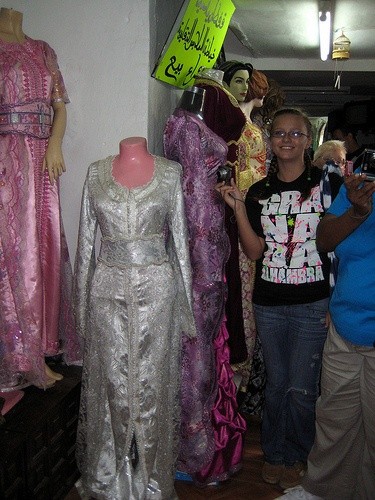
[{"x1": 274, "y1": 484, "x2": 324, "y2": 500}]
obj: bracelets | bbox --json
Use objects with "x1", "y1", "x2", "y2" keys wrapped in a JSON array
[{"x1": 347, "y1": 205, "x2": 371, "y2": 219}]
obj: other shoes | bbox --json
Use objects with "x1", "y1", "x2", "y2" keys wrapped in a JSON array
[
  {"x1": 280, "y1": 466, "x2": 306, "y2": 488},
  {"x1": 262, "y1": 463, "x2": 284, "y2": 484}
]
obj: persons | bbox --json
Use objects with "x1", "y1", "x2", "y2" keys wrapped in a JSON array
[
  {"x1": 0, "y1": 6, "x2": 77, "y2": 394},
  {"x1": 215, "y1": 105, "x2": 338, "y2": 490},
  {"x1": 268, "y1": 156, "x2": 375, "y2": 500},
  {"x1": 236, "y1": 70, "x2": 273, "y2": 188},
  {"x1": 214, "y1": 60, "x2": 269, "y2": 396},
  {"x1": 330, "y1": 118, "x2": 364, "y2": 171},
  {"x1": 73, "y1": 135, "x2": 197, "y2": 500},
  {"x1": 193, "y1": 68, "x2": 249, "y2": 367},
  {"x1": 312, "y1": 141, "x2": 346, "y2": 178},
  {"x1": 250, "y1": 77, "x2": 287, "y2": 174},
  {"x1": 164, "y1": 88, "x2": 245, "y2": 489}
]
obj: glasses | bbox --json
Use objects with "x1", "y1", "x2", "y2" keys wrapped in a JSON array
[{"x1": 271, "y1": 129, "x2": 309, "y2": 137}]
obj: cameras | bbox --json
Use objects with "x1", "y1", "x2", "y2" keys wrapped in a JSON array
[
  {"x1": 360, "y1": 150, "x2": 375, "y2": 182},
  {"x1": 217, "y1": 166, "x2": 232, "y2": 186}
]
